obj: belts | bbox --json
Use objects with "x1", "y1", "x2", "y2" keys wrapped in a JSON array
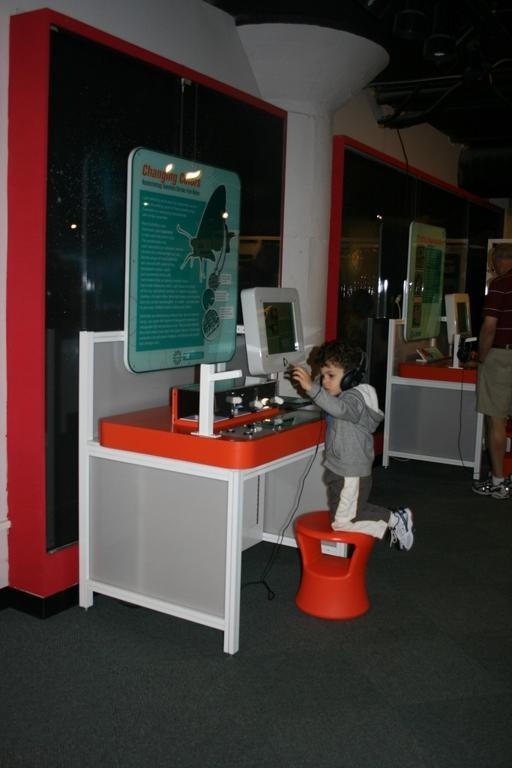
[{"x1": 489, "y1": 342, "x2": 512, "y2": 350}]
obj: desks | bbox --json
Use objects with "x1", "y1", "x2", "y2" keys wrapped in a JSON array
[
  {"x1": 380, "y1": 317, "x2": 483, "y2": 481},
  {"x1": 78, "y1": 326, "x2": 347, "y2": 657}
]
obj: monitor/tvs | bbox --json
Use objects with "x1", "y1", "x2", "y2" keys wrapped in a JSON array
[
  {"x1": 240, "y1": 286, "x2": 306, "y2": 376},
  {"x1": 444, "y1": 293, "x2": 472, "y2": 345}
]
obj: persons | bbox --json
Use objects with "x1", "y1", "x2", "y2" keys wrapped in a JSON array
[
  {"x1": 293, "y1": 339, "x2": 416, "y2": 554},
  {"x1": 471, "y1": 243, "x2": 511, "y2": 500}
]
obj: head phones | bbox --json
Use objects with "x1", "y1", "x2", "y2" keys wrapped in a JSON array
[
  {"x1": 457, "y1": 336, "x2": 472, "y2": 363},
  {"x1": 340, "y1": 348, "x2": 367, "y2": 391}
]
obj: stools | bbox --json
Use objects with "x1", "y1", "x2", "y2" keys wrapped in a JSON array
[{"x1": 293, "y1": 510, "x2": 375, "y2": 621}]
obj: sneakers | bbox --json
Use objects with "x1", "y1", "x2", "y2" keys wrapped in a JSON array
[
  {"x1": 471, "y1": 478, "x2": 512, "y2": 500},
  {"x1": 389, "y1": 507, "x2": 415, "y2": 552}
]
